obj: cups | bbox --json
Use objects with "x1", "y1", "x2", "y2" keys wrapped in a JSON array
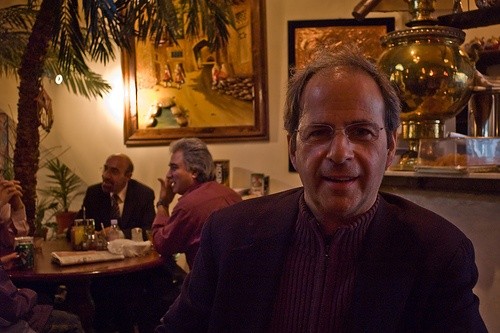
[
  {"x1": 71, "y1": 218, "x2": 151, "y2": 252},
  {"x1": 33, "y1": 238, "x2": 43, "y2": 254}
]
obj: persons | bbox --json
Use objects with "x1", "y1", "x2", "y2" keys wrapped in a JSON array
[
  {"x1": 154, "y1": 46, "x2": 490, "y2": 333},
  {"x1": 0, "y1": 167, "x2": 87, "y2": 333},
  {"x1": 151, "y1": 137, "x2": 243, "y2": 272},
  {"x1": 66, "y1": 153, "x2": 155, "y2": 333}
]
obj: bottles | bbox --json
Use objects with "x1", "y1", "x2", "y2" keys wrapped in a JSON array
[{"x1": 109, "y1": 220, "x2": 122, "y2": 241}]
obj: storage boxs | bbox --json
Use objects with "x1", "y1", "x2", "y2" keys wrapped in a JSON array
[
  {"x1": 249, "y1": 174, "x2": 270, "y2": 197},
  {"x1": 213, "y1": 161, "x2": 230, "y2": 187}
]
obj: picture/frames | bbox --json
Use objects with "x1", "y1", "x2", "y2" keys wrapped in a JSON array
[
  {"x1": 286, "y1": 17, "x2": 395, "y2": 173},
  {"x1": 119, "y1": 0, "x2": 270, "y2": 147}
]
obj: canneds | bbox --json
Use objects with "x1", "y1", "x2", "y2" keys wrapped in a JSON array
[{"x1": 13, "y1": 236, "x2": 34, "y2": 271}]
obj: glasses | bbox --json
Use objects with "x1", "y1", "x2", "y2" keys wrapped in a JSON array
[{"x1": 295, "y1": 124, "x2": 386, "y2": 145}]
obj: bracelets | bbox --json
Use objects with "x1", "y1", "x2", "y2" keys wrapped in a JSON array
[{"x1": 157, "y1": 201, "x2": 169, "y2": 208}]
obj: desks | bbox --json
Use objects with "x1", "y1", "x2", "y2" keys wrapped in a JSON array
[{"x1": 6, "y1": 238, "x2": 166, "y2": 333}]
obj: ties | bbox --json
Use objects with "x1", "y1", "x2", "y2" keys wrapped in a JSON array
[{"x1": 111, "y1": 194, "x2": 120, "y2": 220}]
